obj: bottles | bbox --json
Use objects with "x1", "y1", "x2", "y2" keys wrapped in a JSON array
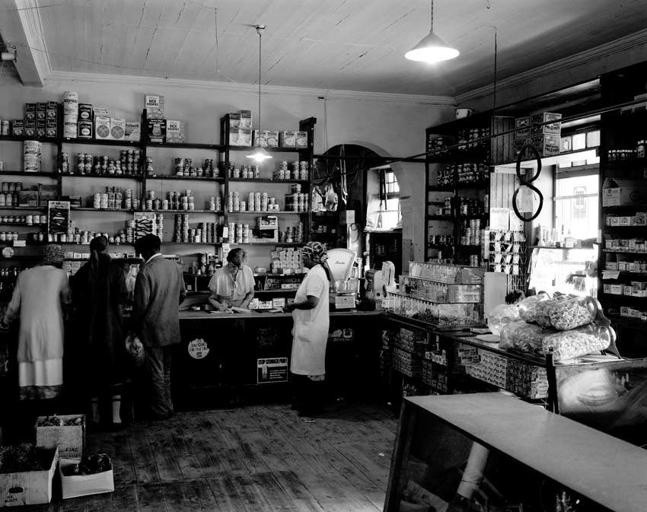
[
  {"x1": 606, "y1": 139, "x2": 646, "y2": 160},
  {"x1": 426, "y1": 125, "x2": 490, "y2": 246}
]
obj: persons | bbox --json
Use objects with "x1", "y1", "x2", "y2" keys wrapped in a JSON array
[
  {"x1": 128, "y1": 232, "x2": 188, "y2": 422},
  {"x1": 283, "y1": 241, "x2": 335, "y2": 419},
  {"x1": 80, "y1": 235, "x2": 128, "y2": 425},
  {"x1": 3, "y1": 242, "x2": 74, "y2": 401},
  {"x1": 208, "y1": 248, "x2": 258, "y2": 311}
]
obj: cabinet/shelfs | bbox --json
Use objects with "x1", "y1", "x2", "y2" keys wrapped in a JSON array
[
  {"x1": 381, "y1": 314, "x2": 647, "y2": 450},
  {"x1": 383, "y1": 386, "x2": 645, "y2": 511},
  {"x1": 368, "y1": 230, "x2": 400, "y2": 272}
]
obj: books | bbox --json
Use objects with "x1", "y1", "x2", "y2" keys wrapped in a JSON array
[{"x1": 214, "y1": 305, "x2": 253, "y2": 316}]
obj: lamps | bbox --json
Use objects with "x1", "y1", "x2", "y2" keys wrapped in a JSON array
[
  {"x1": 402, "y1": 0, "x2": 459, "y2": 71},
  {"x1": 241, "y1": 16, "x2": 272, "y2": 162}
]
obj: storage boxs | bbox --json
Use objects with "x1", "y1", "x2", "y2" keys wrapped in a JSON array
[{"x1": 0, "y1": 413, "x2": 117, "y2": 511}]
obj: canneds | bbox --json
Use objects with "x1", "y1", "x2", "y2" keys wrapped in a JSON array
[
  {"x1": 74, "y1": 227, "x2": 134, "y2": 245},
  {"x1": 273, "y1": 160, "x2": 308, "y2": 180},
  {"x1": 233, "y1": 164, "x2": 259, "y2": 180},
  {"x1": 0, "y1": 215, "x2": 46, "y2": 226},
  {"x1": 229, "y1": 192, "x2": 280, "y2": 211},
  {"x1": 62, "y1": 148, "x2": 140, "y2": 175},
  {"x1": 230, "y1": 222, "x2": 249, "y2": 244},
  {"x1": 0, "y1": 181, "x2": 22, "y2": 206},
  {"x1": 285, "y1": 183, "x2": 309, "y2": 212},
  {"x1": 92, "y1": 186, "x2": 137, "y2": 211},
  {"x1": 281, "y1": 221, "x2": 304, "y2": 243},
  {"x1": 146, "y1": 190, "x2": 195, "y2": 210},
  {"x1": 175, "y1": 213, "x2": 218, "y2": 243},
  {"x1": 175, "y1": 156, "x2": 220, "y2": 178},
  {"x1": 209, "y1": 196, "x2": 222, "y2": 210}
]
